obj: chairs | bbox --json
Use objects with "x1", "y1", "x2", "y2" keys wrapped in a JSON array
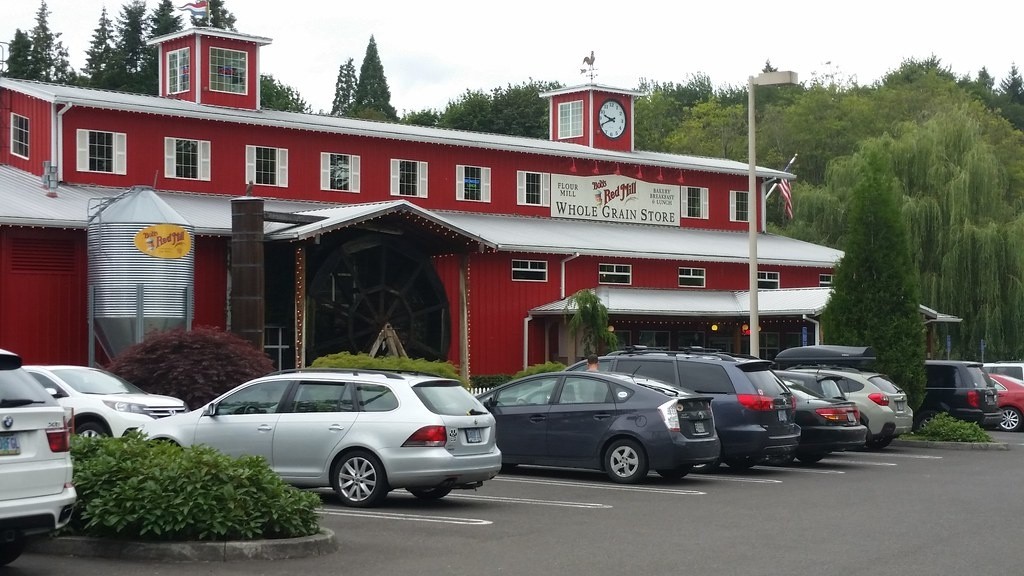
[
  {"x1": 336, "y1": 388, "x2": 350, "y2": 410},
  {"x1": 563, "y1": 385, "x2": 575, "y2": 404},
  {"x1": 298, "y1": 391, "x2": 317, "y2": 410}
]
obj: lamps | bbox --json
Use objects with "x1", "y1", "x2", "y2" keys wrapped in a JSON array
[
  {"x1": 567, "y1": 158, "x2": 576, "y2": 173},
  {"x1": 592, "y1": 161, "x2": 599, "y2": 175},
  {"x1": 637, "y1": 166, "x2": 642, "y2": 178},
  {"x1": 608, "y1": 326, "x2": 615, "y2": 332},
  {"x1": 614, "y1": 163, "x2": 621, "y2": 175},
  {"x1": 742, "y1": 324, "x2": 748, "y2": 335},
  {"x1": 657, "y1": 167, "x2": 663, "y2": 181},
  {"x1": 678, "y1": 170, "x2": 684, "y2": 183}
]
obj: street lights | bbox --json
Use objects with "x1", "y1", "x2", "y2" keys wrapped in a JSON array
[{"x1": 747, "y1": 69, "x2": 799, "y2": 358}]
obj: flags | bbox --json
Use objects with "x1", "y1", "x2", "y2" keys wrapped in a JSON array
[{"x1": 779, "y1": 162, "x2": 793, "y2": 220}]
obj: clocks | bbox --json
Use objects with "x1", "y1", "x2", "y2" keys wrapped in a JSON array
[{"x1": 598, "y1": 98, "x2": 627, "y2": 139}]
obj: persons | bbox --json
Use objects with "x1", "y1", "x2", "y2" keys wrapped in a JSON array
[{"x1": 575, "y1": 354, "x2": 608, "y2": 402}]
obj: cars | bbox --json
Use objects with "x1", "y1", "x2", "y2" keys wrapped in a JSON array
[
  {"x1": 21, "y1": 364, "x2": 190, "y2": 439},
  {"x1": 988, "y1": 374, "x2": 1024, "y2": 432},
  {"x1": 763, "y1": 378, "x2": 868, "y2": 466},
  {"x1": 473, "y1": 369, "x2": 723, "y2": 484}
]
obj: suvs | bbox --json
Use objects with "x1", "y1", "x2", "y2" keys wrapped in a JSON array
[
  {"x1": 981, "y1": 360, "x2": 1024, "y2": 387},
  {"x1": 555, "y1": 344, "x2": 804, "y2": 475},
  {"x1": 0, "y1": 349, "x2": 77, "y2": 569},
  {"x1": 132, "y1": 364, "x2": 502, "y2": 508},
  {"x1": 774, "y1": 345, "x2": 914, "y2": 453},
  {"x1": 915, "y1": 360, "x2": 1003, "y2": 435}
]
